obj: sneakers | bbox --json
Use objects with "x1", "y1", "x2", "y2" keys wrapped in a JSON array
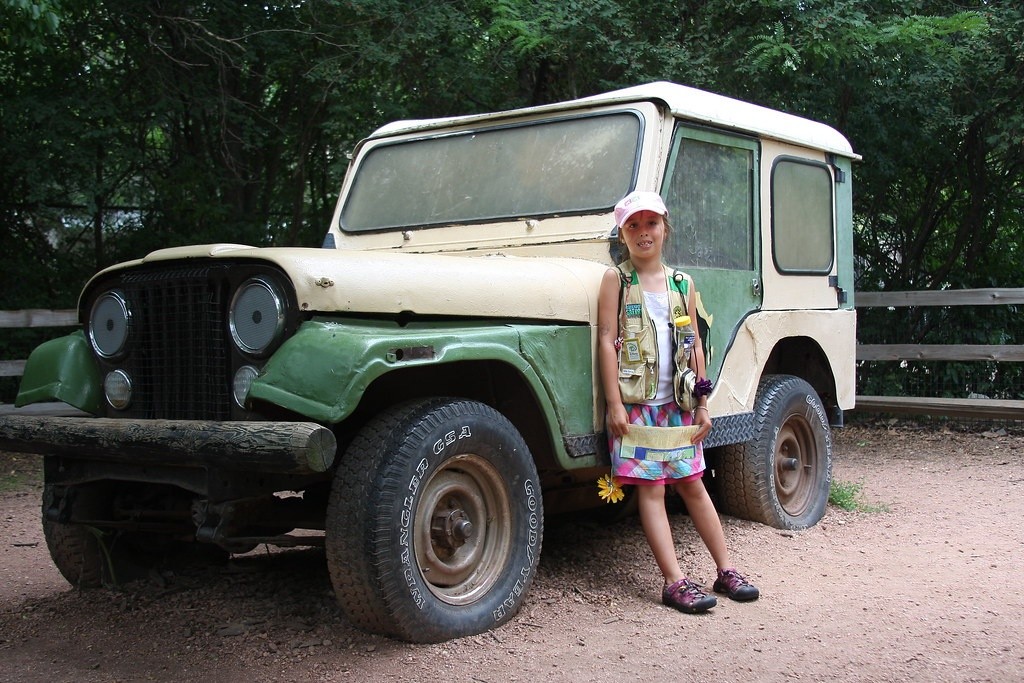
[
  {"x1": 662, "y1": 577, "x2": 717, "y2": 614},
  {"x1": 713, "y1": 569, "x2": 759, "y2": 600}
]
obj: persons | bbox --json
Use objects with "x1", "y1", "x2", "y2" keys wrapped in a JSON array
[{"x1": 596, "y1": 192, "x2": 761, "y2": 613}]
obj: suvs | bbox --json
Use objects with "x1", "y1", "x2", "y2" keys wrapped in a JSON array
[{"x1": 15, "y1": 81, "x2": 871, "y2": 645}]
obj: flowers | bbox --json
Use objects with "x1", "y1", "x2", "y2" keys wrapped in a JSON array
[{"x1": 597, "y1": 474, "x2": 626, "y2": 502}]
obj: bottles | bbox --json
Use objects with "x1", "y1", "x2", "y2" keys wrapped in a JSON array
[{"x1": 674, "y1": 317, "x2": 696, "y2": 348}]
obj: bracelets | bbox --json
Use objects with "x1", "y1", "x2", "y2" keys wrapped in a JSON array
[{"x1": 697, "y1": 405, "x2": 709, "y2": 412}]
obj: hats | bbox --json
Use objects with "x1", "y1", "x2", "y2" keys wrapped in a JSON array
[{"x1": 614, "y1": 191, "x2": 669, "y2": 229}]
